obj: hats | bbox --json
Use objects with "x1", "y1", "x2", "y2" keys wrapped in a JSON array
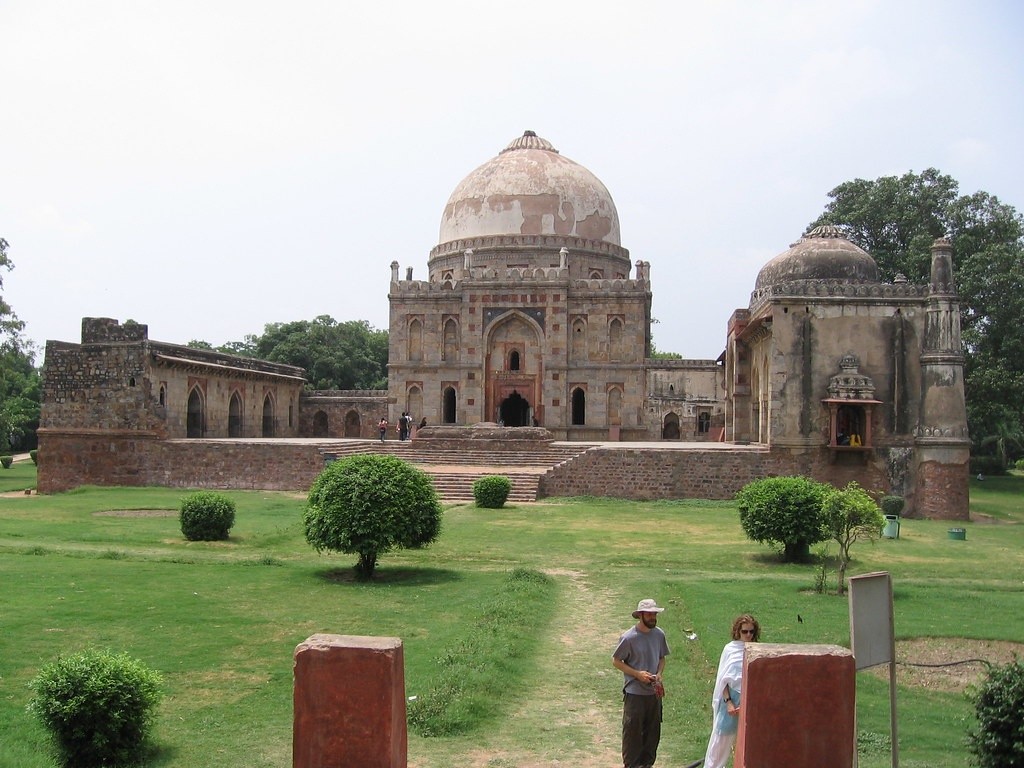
[{"x1": 632, "y1": 599, "x2": 664, "y2": 618}]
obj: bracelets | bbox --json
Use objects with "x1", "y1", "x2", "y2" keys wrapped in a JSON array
[{"x1": 723, "y1": 698, "x2": 731, "y2": 703}]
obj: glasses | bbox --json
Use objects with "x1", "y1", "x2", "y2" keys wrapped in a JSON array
[{"x1": 740, "y1": 630, "x2": 755, "y2": 634}]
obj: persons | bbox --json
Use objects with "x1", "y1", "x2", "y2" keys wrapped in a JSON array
[
  {"x1": 851, "y1": 430, "x2": 861, "y2": 446},
  {"x1": 420, "y1": 417, "x2": 428, "y2": 427},
  {"x1": 399, "y1": 412, "x2": 412, "y2": 441},
  {"x1": 612, "y1": 598, "x2": 670, "y2": 768},
  {"x1": 703, "y1": 614, "x2": 761, "y2": 768},
  {"x1": 379, "y1": 418, "x2": 388, "y2": 442},
  {"x1": 838, "y1": 428, "x2": 850, "y2": 445}
]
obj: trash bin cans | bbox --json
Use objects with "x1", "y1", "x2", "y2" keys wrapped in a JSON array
[{"x1": 882, "y1": 514, "x2": 897, "y2": 539}]
